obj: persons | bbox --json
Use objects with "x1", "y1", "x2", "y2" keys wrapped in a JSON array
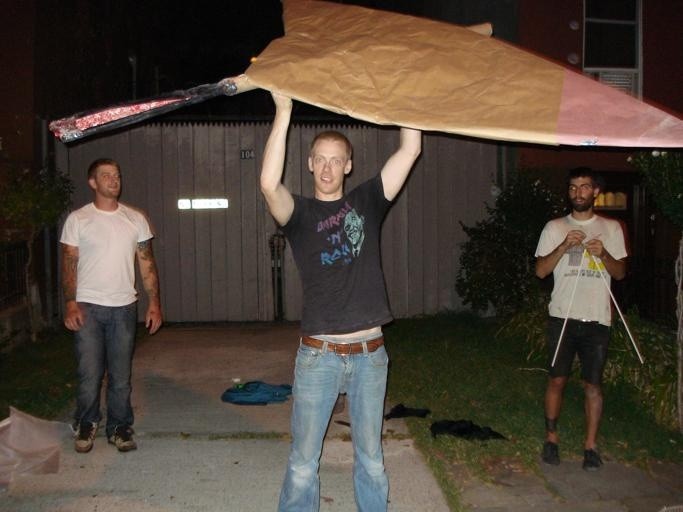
[
  {"x1": 254, "y1": 78, "x2": 423, "y2": 511},
  {"x1": 56, "y1": 157, "x2": 166, "y2": 455},
  {"x1": 530, "y1": 164, "x2": 633, "y2": 468}
]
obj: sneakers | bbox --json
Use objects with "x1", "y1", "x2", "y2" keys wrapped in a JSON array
[
  {"x1": 73, "y1": 428, "x2": 95, "y2": 452},
  {"x1": 543, "y1": 441, "x2": 559, "y2": 464},
  {"x1": 583, "y1": 448, "x2": 602, "y2": 471},
  {"x1": 110, "y1": 426, "x2": 137, "y2": 452}
]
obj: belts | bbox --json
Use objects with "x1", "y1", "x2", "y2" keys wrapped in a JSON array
[{"x1": 302, "y1": 336, "x2": 384, "y2": 355}]
